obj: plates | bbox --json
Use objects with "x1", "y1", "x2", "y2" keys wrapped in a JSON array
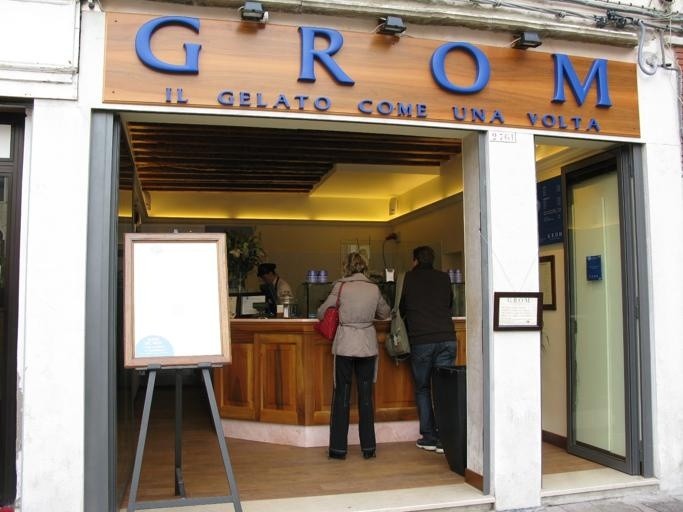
[{"x1": 304, "y1": 312, "x2": 317, "y2": 319}]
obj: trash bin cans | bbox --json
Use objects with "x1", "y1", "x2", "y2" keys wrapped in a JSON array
[{"x1": 430, "y1": 365, "x2": 466, "y2": 477}]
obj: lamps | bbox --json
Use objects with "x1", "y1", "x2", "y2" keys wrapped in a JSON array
[
  {"x1": 238, "y1": 3, "x2": 270, "y2": 25},
  {"x1": 375, "y1": 16, "x2": 407, "y2": 35},
  {"x1": 511, "y1": 31, "x2": 542, "y2": 50}
]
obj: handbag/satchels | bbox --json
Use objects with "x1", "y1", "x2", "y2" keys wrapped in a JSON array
[
  {"x1": 312, "y1": 306, "x2": 338, "y2": 340},
  {"x1": 385, "y1": 310, "x2": 411, "y2": 357}
]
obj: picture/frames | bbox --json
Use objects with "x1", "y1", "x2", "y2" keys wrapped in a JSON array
[{"x1": 538, "y1": 254, "x2": 556, "y2": 310}]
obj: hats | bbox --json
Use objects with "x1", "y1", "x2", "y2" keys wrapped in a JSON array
[{"x1": 257, "y1": 263, "x2": 275, "y2": 277}]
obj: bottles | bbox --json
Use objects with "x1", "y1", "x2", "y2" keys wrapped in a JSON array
[
  {"x1": 448, "y1": 268, "x2": 462, "y2": 284},
  {"x1": 307, "y1": 269, "x2": 329, "y2": 284},
  {"x1": 282, "y1": 290, "x2": 291, "y2": 318}
]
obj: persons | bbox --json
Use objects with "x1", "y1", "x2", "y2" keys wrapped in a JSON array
[
  {"x1": 258, "y1": 262, "x2": 295, "y2": 316},
  {"x1": 317, "y1": 252, "x2": 392, "y2": 460},
  {"x1": 391, "y1": 246, "x2": 458, "y2": 454}
]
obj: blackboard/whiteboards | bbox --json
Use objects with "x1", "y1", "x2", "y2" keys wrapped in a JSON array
[{"x1": 122, "y1": 232, "x2": 233, "y2": 369}]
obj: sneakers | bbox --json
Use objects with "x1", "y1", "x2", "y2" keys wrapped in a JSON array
[
  {"x1": 364, "y1": 450, "x2": 375, "y2": 458},
  {"x1": 329, "y1": 451, "x2": 345, "y2": 459},
  {"x1": 416, "y1": 439, "x2": 445, "y2": 454}
]
